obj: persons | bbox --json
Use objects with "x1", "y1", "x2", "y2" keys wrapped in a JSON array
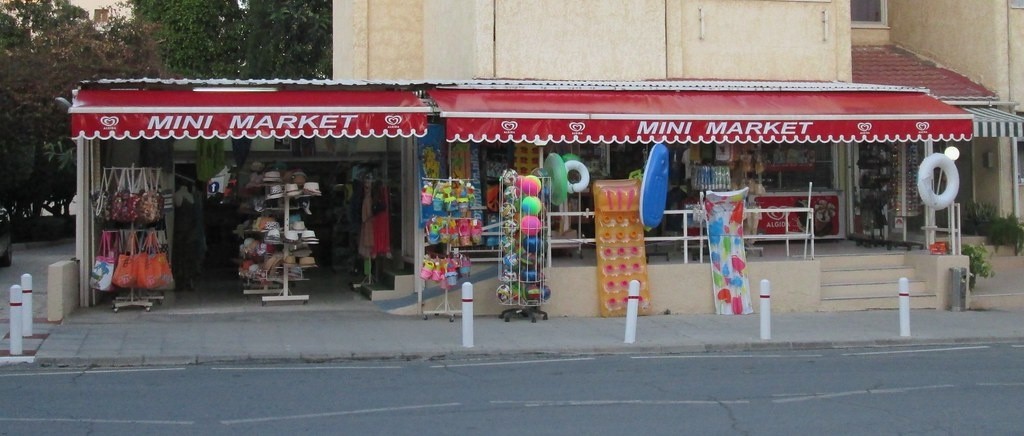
[
  {"x1": 174, "y1": 186, "x2": 207, "y2": 291},
  {"x1": 358, "y1": 160, "x2": 392, "y2": 282}
]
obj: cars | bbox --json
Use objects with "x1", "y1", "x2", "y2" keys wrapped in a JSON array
[{"x1": 0, "y1": 204, "x2": 13, "y2": 266}]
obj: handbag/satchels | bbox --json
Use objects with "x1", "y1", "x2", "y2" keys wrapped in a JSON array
[{"x1": 89, "y1": 168, "x2": 175, "y2": 291}]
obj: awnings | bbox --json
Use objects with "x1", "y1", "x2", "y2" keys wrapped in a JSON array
[
  {"x1": 426, "y1": 91, "x2": 975, "y2": 146},
  {"x1": 68, "y1": 84, "x2": 432, "y2": 140},
  {"x1": 961, "y1": 108, "x2": 1024, "y2": 138}
]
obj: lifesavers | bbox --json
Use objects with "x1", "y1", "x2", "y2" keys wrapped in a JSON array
[
  {"x1": 544, "y1": 152, "x2": 590, "y2": 206},
  {"x1": 639, "y1": 144, "x2": 669, "y2": 228},
  {"x1": 918, "y1": 152, "x2": 960, "y2": 210}
]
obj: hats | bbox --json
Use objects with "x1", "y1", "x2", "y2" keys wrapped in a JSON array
[{"x1": 239, "y1": 171, "x2": 321, "y2": 278}]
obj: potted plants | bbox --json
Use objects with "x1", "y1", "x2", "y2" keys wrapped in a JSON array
[{"x1": 962, "y1": 201, "x2": 996, "y2": 236}]
